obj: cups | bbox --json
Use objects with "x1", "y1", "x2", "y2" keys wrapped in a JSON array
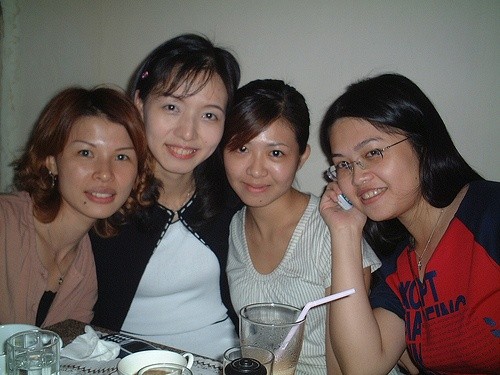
[
  {"x1": 117, "y1": 350, "x2": 193, "y2": 375},
  {"x1": 238, "y1": 302, "x2": 307, "y2": 375},
  {"x1": 5, "y1": 329, "x2": 61, "y2": 375},
  {"x1": 136, "y1": 362, "x2": 193, "y2": 375},
  {"x1": 0, "y1": 323, "x2": 62, "y2": 375},
  {"x1": 222, "y1": 345, "x2": 275, "y2": 375}
]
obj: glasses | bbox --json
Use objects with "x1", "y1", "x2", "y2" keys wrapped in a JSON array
[{"x1": 327, "y1": 134, "x2": 414, "y2": 181}]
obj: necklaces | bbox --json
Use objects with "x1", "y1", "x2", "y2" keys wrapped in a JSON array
[
  {"x1": 46, "y1": 228, "x2": 78, "y2": 286},
  {"x1": 414, "y1": 208, "x2": 444, "y2": 270}
]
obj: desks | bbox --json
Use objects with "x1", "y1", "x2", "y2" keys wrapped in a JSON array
[{"x1": 37, "y1": 318, "x2": 224, "y2": 375}]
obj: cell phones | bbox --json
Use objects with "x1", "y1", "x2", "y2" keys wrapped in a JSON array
[
  {"x1": 98, "y1": 333, "x2": 159, "y2": 358},
  {"x1": 337, "y1": 194, "x2": 353, "y2": 210}
]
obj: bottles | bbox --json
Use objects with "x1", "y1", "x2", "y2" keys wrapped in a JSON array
[{"x1": 224, "y1": 357, "x2": 268, "y2": 375}]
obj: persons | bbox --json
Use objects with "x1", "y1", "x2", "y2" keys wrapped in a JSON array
[
  {"x1": 320, "y1": 74, "x2": 500, "y2": 375},
  {"x1": 0, "y1": 87, "x2": 164, "y2": 329},
  {"x1": 220, "y1": 79, "x2": 402, "y2": 375},
  {"x1": 88, "y1": 34, "x2": 245, "y2": 363}
]
